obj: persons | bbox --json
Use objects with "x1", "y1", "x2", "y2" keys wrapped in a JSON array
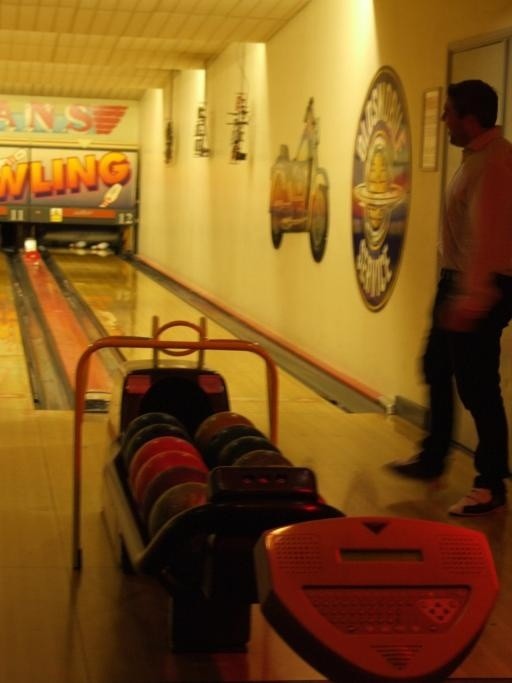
[{"x1": 384, "y1": 79, "x2": 511, "y2": 518}]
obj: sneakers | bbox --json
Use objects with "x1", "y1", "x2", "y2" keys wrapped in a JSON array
[
  {"x1": 388, "y1": 457, "x2": 448, "y2": 480},
  {"x1": 448, "y1": 473, "x2": 508, "y2": 517}
]
